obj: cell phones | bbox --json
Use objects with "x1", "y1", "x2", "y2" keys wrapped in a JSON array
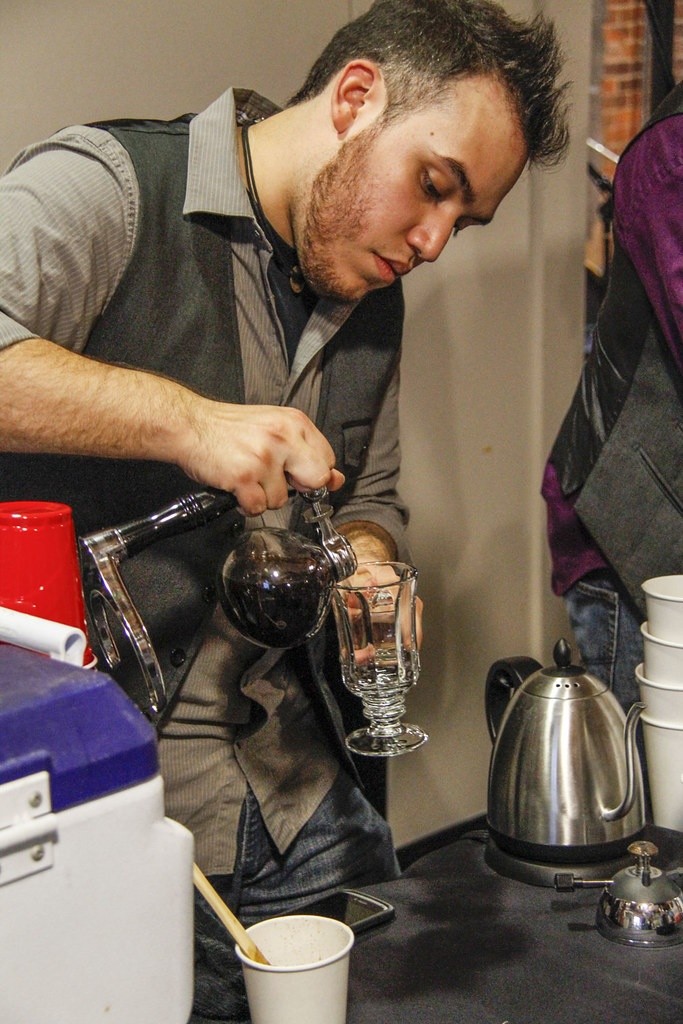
[{"x1": 260, "y1": 888, "x2": 395, "y2": 934}]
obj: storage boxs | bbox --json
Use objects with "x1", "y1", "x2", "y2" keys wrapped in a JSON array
[{"x1": 0, "y1": 640, "x2": 195, "y2": 1024}]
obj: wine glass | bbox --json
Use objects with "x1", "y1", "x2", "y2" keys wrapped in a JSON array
[{"x1": 332, "y1": 562, "x2": 428, "y2": 757}]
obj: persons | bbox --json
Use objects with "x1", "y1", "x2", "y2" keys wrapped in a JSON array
[
  {"x1": 539, "y1": 83, "x2": 683, "y2": 713},
  {"x1": 2, "y1": 2, "x2": 573, "y2": 1024}
]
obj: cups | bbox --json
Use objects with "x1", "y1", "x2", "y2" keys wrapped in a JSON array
[
  {"x1": 234, "y1": 914, "x2": 354, "y2": 1024},
  {"x1": 1, "y1": 501, "x2": 97, "y2": 670},
  {"x1": 635, "y1": 574, "x2": 683, "y2": 831}
]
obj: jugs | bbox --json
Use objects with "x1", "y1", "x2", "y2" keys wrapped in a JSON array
[{"x1": 209, "y1": 465, "x2": 360, "y2": 650}]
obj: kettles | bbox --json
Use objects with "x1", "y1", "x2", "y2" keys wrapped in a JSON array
[{"x1": 484, "y1": 637, "x2": 651, "y2": 891}]
